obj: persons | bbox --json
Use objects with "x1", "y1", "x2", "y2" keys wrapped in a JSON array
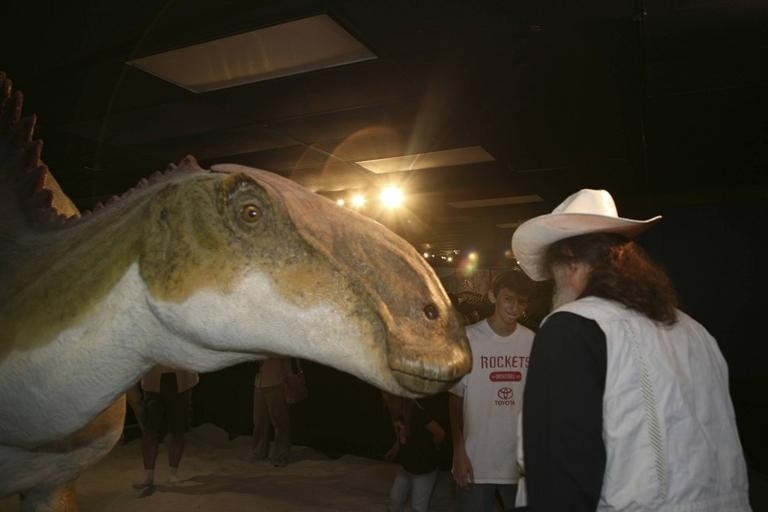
[
  {"x1": 130, "y1": 369, "x2": 201, "y2": 490},
  {"x1": 449, "y1": 270, "x2": 535, "y2": 512},
  {"x1": 513, "y1": 231, "x2": 754, "y2": 512},
  {"x1": 379, "y1": 388, "x2": 447, "y2": 512},
  {"x1": 250, "y1": 358, "x2": 310, "y2": 468}
]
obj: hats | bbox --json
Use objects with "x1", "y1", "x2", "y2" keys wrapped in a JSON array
[{"x1": 509, "y1": 188, "x2": 665, "y2": 282}]
